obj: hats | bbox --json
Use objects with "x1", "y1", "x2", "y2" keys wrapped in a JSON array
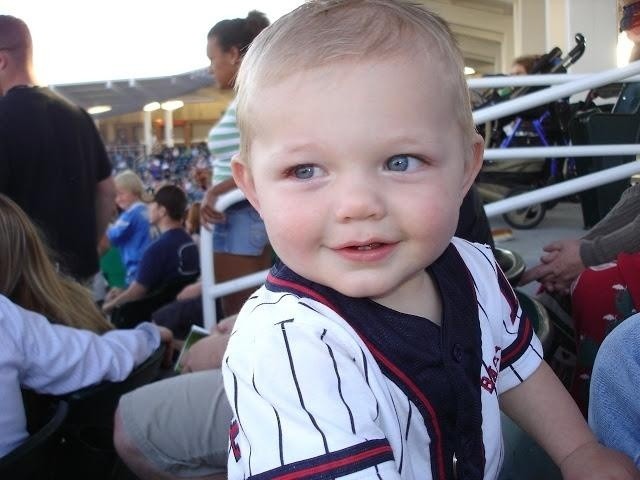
[{"x1": 153, "y1": 184, "x2": 189, "y2": 222}]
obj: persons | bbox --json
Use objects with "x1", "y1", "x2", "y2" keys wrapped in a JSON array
[
  {"x1": 96, "y1": 169, "x2": 158, "y2": 288},
  {"x1": 101, "y1": 185, "x2": 202, "y2": 342},
  {"x1": 198, "y1": 10, "x2": 272, "y2": 317},
  {"x1": 114, "y1": 312, "x2": 238, "y2": 480},
  {"x1": 515, "y1": 184, "x2": 640, "y2": 412},
  {"x1": 0, "y1": 293, "x2": 177, "y2": 480},
  {"x1": 0, "y1": 15, "x2": 118, "y2": 298},
  {"x1": 108, "y1": 142, "x2": 210, "y2": 204},
  {"x1": 497, "y1": 55, "x2": 550, "y2": 132},
  {"x1": 223, "y1": 0, "x2": 640, "y2": 480},
  {"x1": 614, "y1": 0, "x2": 640, "y2": 73},
  {"x1": 0, "y1": 194, "x2": 111, "y2": 334}
]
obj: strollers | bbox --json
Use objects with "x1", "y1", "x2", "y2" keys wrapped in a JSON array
[{"x1": 472, "y1": 33, "x2": 585, "y2": 228}]
